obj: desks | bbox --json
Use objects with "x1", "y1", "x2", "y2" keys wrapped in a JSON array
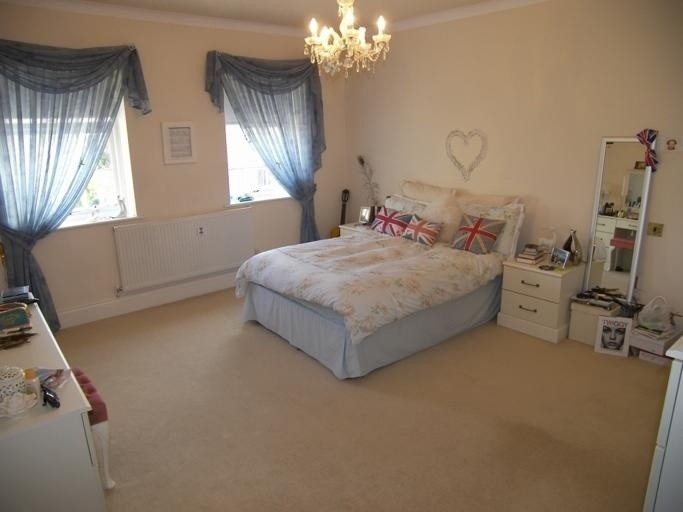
[
  {"x1": 594, "y1": 213, "x2": 639, "y2": 272},
  {"x1": 0, "y1": 292, "x2": 107, "y2": 510}
]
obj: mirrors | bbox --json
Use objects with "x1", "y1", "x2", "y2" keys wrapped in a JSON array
[{"x1": 583, "y1": 135, "x2": 655, "y2": 306}]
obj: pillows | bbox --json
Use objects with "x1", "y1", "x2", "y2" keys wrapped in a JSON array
[{"x1": 370, "y1": 192, "x2": 506, "y2": 256}]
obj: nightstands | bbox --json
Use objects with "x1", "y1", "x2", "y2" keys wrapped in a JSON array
[{"x1": 496, "y1": 257, "x2": 586, "y2": 345}]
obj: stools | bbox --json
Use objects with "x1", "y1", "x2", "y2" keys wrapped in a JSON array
[
  {"x1": 68, "y1": 365, "x2": 115, "y2": 491},
  {"x1": 609, "y1": 237, "x2": 634, "y2": 272}
]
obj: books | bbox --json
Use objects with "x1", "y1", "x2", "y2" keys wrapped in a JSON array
[
  {"x1": 635, "y1": 325, "x2": 676, "y2": 342},
  {"x1": 0, "y1": 285, "x2": 31, "y2": 302},
  {"x1": 518, "y1": 250, "x2": 544, "y2": 260},
  {"x1": 570, "y1": 295, "x2": 618, "y2": 310},
  {"x1": 523, "y1": 244, "x2": 539, "y2": 253},
  {"x1": 516, "y1": 255, "x2": 545, "y2": 265}
]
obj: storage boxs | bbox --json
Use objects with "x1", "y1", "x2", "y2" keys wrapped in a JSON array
[{"x1": 569, "y1": 300, "x2": 622, "y2": 349}]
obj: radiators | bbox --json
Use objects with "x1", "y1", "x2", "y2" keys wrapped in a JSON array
[{"x1": 112, "y1": 206, "x2": 254, "y2": 296}]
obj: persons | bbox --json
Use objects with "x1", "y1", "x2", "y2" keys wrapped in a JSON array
[
  {"x1": 551, "y1": 255, "x2": 557, "y2": 264},
  {"x1": 555, "y1": 255, "x2": 561, "y2": 265},
  {"x1": 666, "y1": 138, "x2": 677, "y2": 151},
  {"x1": 602, "y1": 326, "x2": 626, "y2": 352}
]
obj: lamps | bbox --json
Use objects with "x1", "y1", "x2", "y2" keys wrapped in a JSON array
[{"x1": 301, "y1": 2, "x2": 391, "y2": 84}]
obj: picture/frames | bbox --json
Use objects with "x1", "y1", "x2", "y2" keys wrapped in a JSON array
[
  {"x1": 358, "y1": 206, "x2": 370, "y2": 224},
  {"x1": 160, "y1": 121, "x2": 199, "y2": 165}
]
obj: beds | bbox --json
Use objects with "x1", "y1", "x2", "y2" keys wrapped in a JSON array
[{"x1": 233, "y1": 184, "x2": 525, "y2": 377}]
